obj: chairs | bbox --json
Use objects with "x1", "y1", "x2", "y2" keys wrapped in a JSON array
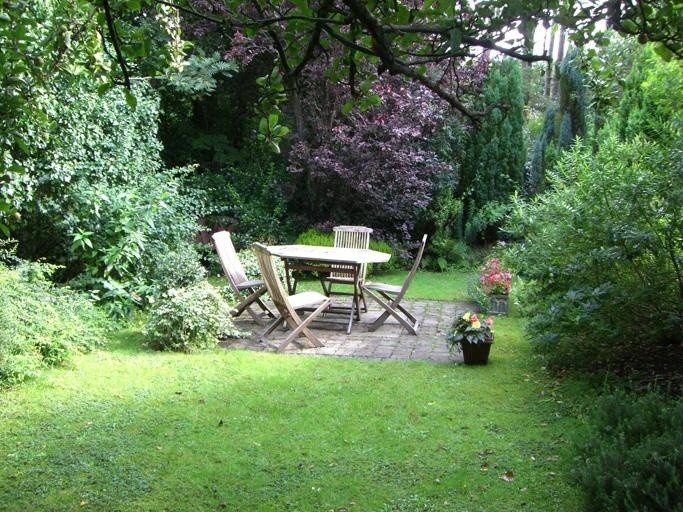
[{"x1": 211, "y1": 224, "x2": 427, "y2": 354}]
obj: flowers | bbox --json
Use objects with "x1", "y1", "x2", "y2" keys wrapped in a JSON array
[
  {"x1": 476, "y1": 256, "x2": 511, "y2": 295},
  {"x1": 445, "y1": 309, "x2": 494, "y2": 361}
]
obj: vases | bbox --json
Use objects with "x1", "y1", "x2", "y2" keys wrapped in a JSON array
[
  {"x1": 489, "y1": 295, "x2": 509, "y2": 315},
  {"x1": 459, "y1": 338, "x2": 493, "y2": 365}
]
